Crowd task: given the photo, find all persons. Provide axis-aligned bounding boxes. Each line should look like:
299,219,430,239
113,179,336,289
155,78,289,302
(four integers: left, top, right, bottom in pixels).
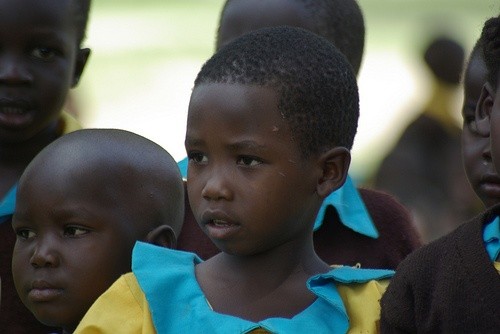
0,125,191,334
55,26,384,334
176,0,420,268
2,0,95,224
379,17,500,334
